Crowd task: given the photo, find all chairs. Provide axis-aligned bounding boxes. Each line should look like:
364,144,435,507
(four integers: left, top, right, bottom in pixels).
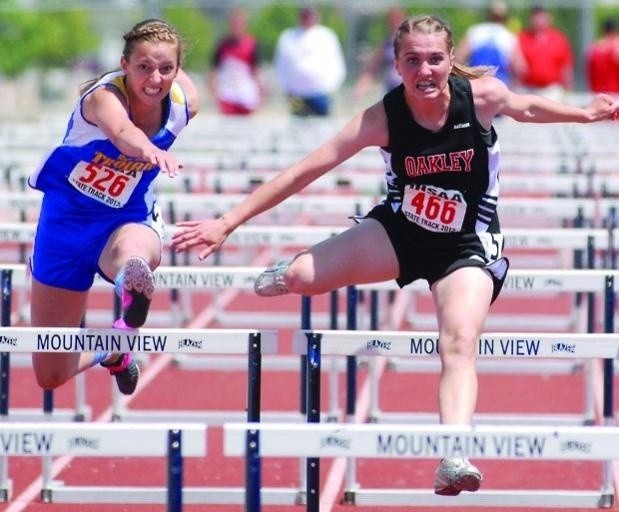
434,457,483,495
120,257,156,327
101,353,139,395
255,260,292,297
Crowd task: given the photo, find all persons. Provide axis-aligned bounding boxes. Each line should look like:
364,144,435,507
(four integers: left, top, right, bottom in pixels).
355,9,412,107
172,18,618,496
454,3,524,117
513,7,572,94
206,7,261,115
30,18,202,398
583,18,618,93
273,6,346,118
